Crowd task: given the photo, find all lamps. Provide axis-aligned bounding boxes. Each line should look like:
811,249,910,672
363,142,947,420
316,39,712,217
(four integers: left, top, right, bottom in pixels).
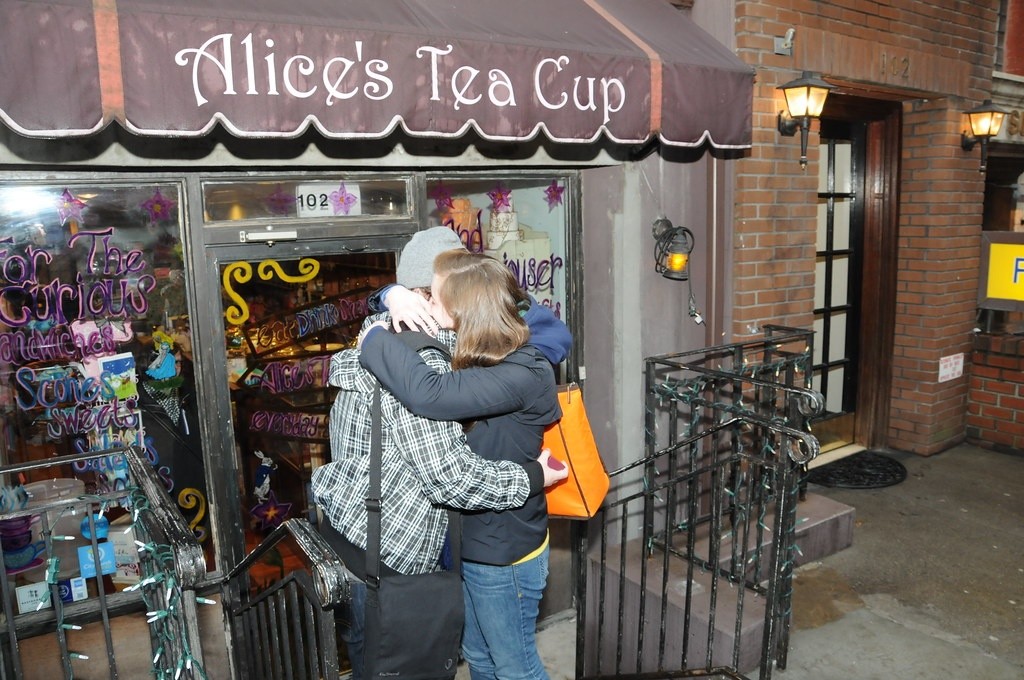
781,71,837,166
644,217,707,281
957,95,1007,152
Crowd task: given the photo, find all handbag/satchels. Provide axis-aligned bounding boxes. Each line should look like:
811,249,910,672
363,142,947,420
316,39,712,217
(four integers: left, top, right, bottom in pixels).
541,381,611,521
360,568,465,680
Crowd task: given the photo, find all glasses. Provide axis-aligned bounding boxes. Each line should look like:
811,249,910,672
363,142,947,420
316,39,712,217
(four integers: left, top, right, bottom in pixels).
175,359,183,367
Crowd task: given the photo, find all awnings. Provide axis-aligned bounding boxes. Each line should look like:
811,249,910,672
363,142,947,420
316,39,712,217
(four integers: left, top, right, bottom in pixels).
0,0,753,149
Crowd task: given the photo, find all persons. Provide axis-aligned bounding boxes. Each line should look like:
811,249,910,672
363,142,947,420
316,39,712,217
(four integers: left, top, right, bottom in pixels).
312,224,573,679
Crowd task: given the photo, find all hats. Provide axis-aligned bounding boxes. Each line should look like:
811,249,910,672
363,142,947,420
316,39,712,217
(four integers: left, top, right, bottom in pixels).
396,226,465,289
